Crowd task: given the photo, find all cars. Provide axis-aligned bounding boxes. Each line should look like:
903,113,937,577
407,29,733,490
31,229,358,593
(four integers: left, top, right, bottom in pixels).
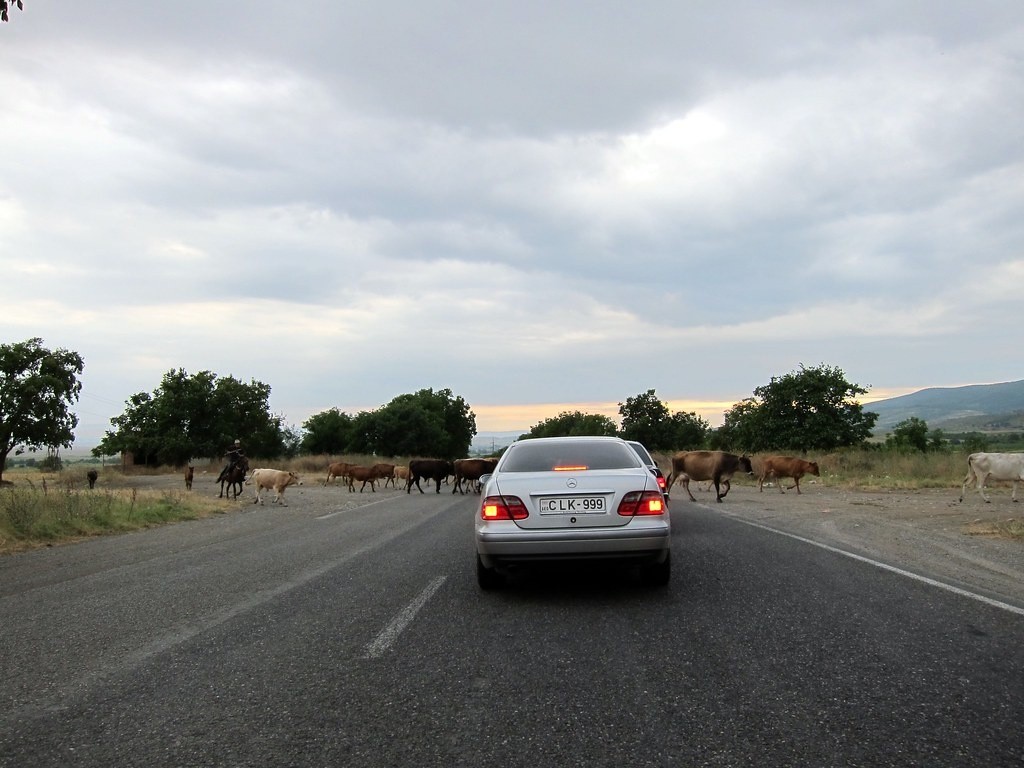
474,436,672,593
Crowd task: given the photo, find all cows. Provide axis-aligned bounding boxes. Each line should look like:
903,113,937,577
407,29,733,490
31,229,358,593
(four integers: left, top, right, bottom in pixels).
959,452,1024,503
244,468,304,507
665,451,754,503
408,459,455,494
394,466,415,490
758,456,820,494
324,463,395,493
452,457,500,496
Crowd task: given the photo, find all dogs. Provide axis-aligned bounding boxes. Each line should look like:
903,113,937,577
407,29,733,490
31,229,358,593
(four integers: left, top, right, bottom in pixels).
185,466,194,490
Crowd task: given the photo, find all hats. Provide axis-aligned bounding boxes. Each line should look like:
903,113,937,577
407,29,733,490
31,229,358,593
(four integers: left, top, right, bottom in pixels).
234,439,240,443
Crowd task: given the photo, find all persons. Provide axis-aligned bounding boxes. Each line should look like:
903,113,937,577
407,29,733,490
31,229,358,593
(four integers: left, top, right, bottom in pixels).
225,439,246,481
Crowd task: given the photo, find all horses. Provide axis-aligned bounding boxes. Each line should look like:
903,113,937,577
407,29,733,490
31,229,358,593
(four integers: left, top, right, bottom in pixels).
219,452,250,501
